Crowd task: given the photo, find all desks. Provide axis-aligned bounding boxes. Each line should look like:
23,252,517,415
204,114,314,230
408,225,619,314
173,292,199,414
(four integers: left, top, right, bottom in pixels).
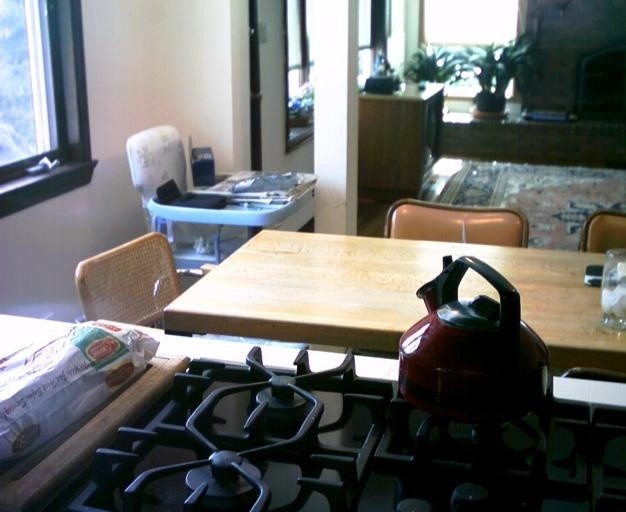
161,228,626,382
149,169,316,272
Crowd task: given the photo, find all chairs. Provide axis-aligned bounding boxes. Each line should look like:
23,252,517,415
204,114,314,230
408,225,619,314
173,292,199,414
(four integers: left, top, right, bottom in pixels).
73,231,218,338
384,198,626,251
126,125,247,273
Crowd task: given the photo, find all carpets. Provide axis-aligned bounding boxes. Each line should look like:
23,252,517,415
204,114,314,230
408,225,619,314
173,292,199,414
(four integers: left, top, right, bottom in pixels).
435,159,625,250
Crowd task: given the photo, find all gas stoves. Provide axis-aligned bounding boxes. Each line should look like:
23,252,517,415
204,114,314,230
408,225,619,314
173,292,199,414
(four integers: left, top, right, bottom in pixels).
70,342,626,512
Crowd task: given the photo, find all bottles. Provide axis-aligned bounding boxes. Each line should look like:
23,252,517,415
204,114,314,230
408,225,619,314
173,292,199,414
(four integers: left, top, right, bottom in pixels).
599,247,626,332
373,48,386,77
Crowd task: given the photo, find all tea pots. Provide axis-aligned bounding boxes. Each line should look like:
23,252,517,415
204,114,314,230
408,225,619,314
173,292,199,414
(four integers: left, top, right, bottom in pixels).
397,254,552,417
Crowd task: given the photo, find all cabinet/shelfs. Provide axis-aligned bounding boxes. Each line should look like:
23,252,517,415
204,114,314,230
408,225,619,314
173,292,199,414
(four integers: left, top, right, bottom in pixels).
356,81,445,203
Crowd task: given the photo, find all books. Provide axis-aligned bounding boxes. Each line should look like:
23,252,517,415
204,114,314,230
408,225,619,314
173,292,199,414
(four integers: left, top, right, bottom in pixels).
205,169,317,209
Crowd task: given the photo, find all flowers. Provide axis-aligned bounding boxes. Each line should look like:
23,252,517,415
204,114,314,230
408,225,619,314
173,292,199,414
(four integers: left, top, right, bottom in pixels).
287,81,314,120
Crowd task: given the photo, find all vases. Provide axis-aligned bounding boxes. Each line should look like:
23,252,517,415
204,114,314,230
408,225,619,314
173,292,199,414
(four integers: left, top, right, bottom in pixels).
289,117,310,127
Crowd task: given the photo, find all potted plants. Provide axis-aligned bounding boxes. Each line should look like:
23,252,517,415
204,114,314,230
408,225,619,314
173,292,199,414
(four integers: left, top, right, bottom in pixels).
468,31,540,121
